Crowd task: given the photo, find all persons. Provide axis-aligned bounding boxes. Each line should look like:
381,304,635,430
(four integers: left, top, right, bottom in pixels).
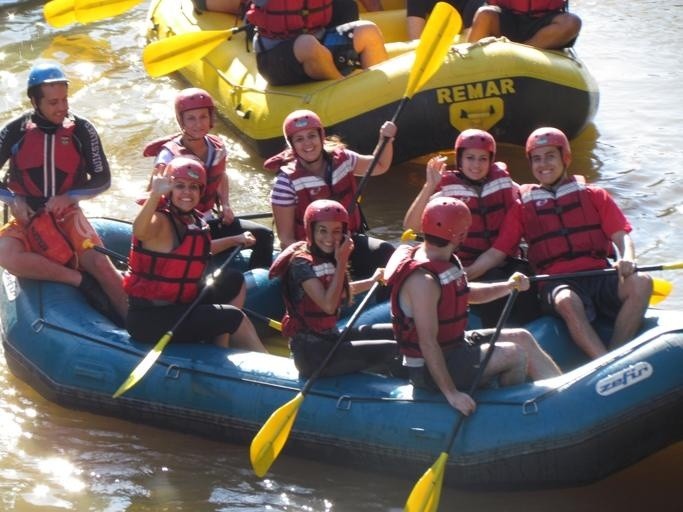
268,105,396,280
146,85,277,274
277,197,402,378
387,193,562,417
0,64,129,324
459,125,655,360
196,0,388,86
402,128,538,330
126,155,271,357
406,0,582,49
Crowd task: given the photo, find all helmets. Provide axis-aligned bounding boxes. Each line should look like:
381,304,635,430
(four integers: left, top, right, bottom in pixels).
454,129,496,166
304,199,350,247
420,196,471,246
525,126,572,166
28,63,70,87
162,156,206,197
175,87,214,128
283,110,326,145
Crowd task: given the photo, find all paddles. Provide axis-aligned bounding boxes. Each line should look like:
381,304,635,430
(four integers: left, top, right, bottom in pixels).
349,2,463,212
113,234,249,399
402,282,520,512
401,229,671,305
141,23,254,80
42,1,77,27
73,1,145,24
249,230,417,478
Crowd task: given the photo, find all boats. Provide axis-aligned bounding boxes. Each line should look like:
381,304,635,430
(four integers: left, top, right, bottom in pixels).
0,214,682,493
150,2,598,158
